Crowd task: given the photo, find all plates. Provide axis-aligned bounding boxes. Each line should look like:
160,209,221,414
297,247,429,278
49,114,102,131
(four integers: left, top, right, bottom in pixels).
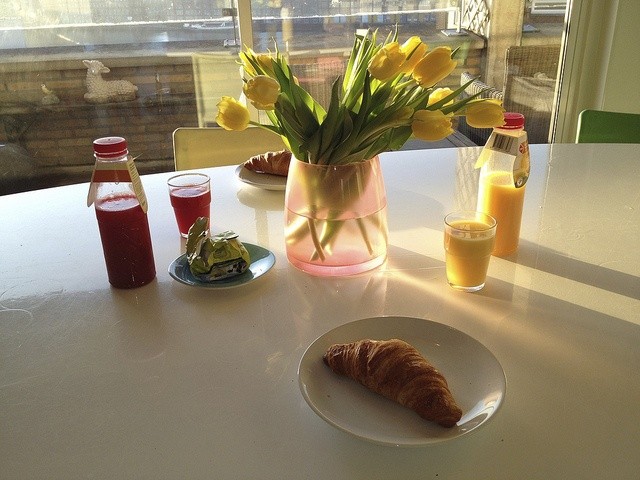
297,315,507,449
168,242,276,290
233,164,286,191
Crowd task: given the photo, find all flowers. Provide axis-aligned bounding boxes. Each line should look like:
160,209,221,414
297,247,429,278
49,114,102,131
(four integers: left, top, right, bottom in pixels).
216,23,506,262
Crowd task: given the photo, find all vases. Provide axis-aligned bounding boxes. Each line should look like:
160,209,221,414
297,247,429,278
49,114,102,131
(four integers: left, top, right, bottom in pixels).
283,153,389,278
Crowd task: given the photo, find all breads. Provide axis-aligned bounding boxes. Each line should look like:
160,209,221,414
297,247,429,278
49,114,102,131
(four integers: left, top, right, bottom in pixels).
239,148,292,177
323,339,462,428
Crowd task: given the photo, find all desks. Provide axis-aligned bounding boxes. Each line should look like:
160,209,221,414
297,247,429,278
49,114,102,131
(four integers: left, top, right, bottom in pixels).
399,130,479,148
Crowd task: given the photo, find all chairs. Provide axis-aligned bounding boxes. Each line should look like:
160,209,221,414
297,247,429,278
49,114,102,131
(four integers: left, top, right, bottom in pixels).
576,109,640,144
173,126,289,171
457,73,502,145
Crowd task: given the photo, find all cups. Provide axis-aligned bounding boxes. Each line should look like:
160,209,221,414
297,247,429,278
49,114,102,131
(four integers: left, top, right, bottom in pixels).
444,210,497,292
167,173,211,238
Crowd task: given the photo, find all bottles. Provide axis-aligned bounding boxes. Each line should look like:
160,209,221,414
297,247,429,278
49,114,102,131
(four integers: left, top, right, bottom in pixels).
93,137,156,289
475,112,531,258
